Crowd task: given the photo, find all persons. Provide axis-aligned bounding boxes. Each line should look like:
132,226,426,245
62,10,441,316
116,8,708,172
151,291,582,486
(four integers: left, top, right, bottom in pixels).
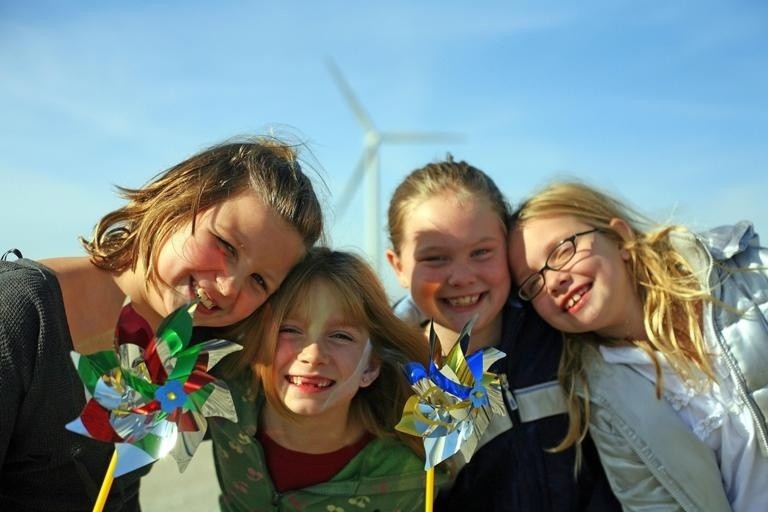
0,133,323,512
179,241,450,512
382,156,623,512
503,178,768,511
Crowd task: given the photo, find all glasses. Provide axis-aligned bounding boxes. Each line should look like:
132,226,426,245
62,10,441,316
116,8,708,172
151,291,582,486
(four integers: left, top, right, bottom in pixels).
514,226,602,302
